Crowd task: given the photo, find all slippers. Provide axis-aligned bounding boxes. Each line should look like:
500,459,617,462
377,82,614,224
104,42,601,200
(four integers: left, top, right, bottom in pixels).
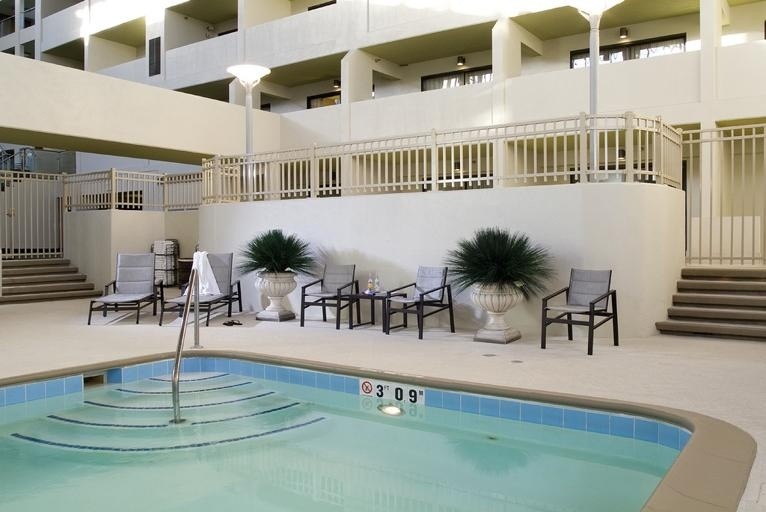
223,320,242,326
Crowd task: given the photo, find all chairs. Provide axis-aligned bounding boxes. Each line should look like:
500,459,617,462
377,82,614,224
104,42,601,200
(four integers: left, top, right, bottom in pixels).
296,260,458,342
540,266,620,357
87,250,243,327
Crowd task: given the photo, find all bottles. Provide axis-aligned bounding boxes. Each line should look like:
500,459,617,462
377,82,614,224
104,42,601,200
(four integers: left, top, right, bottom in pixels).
374,272,380,291
368,273,373,291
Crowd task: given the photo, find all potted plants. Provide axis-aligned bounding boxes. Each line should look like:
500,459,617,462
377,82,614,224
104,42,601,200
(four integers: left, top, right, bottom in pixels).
232,228,324,324
438,226,559,345
